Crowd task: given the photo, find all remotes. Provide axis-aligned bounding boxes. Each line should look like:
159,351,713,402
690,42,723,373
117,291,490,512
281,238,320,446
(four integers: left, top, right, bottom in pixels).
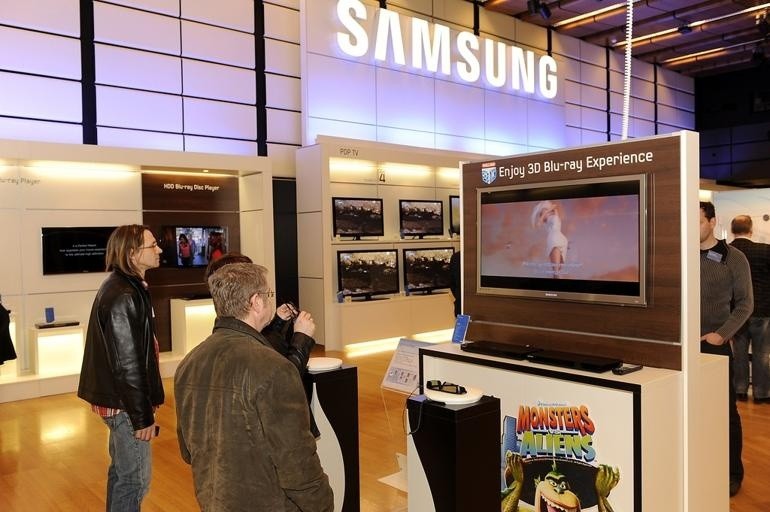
612,364,645,375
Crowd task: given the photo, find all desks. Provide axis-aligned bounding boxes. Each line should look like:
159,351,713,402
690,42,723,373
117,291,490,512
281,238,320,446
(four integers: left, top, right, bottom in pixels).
170,297,220,356
29,326,86,373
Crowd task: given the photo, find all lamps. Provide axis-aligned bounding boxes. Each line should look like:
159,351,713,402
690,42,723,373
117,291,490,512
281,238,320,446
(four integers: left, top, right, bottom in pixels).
756,11,770,40
751,42,764,60
527,0,551,20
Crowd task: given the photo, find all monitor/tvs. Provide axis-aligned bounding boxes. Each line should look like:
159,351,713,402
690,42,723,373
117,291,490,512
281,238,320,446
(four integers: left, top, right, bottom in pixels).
404,247,455,294
449,194,460,234
475,171,650,308
40,225,116,275
332,196,384,241
159,223,228,269
399,198,444,238
337,248,399,301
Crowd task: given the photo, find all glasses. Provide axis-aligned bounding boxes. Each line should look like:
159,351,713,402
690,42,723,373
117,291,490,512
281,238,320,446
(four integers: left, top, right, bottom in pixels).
259,289,274,298
285,300,300,317
137,240,158,250
426,379,467,395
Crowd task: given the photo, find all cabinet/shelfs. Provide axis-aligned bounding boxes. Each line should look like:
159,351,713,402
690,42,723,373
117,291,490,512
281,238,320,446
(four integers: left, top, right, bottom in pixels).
296,135,498,352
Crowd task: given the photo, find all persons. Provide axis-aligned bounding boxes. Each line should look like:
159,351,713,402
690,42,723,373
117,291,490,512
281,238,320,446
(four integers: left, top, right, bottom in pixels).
0,296,17,370
177,231,224,266
529,199,569,280
200,250,324,440
75,220,167,512
727,210,770,404
697,198,756,497
171,261,336,512
447,248,464,317
